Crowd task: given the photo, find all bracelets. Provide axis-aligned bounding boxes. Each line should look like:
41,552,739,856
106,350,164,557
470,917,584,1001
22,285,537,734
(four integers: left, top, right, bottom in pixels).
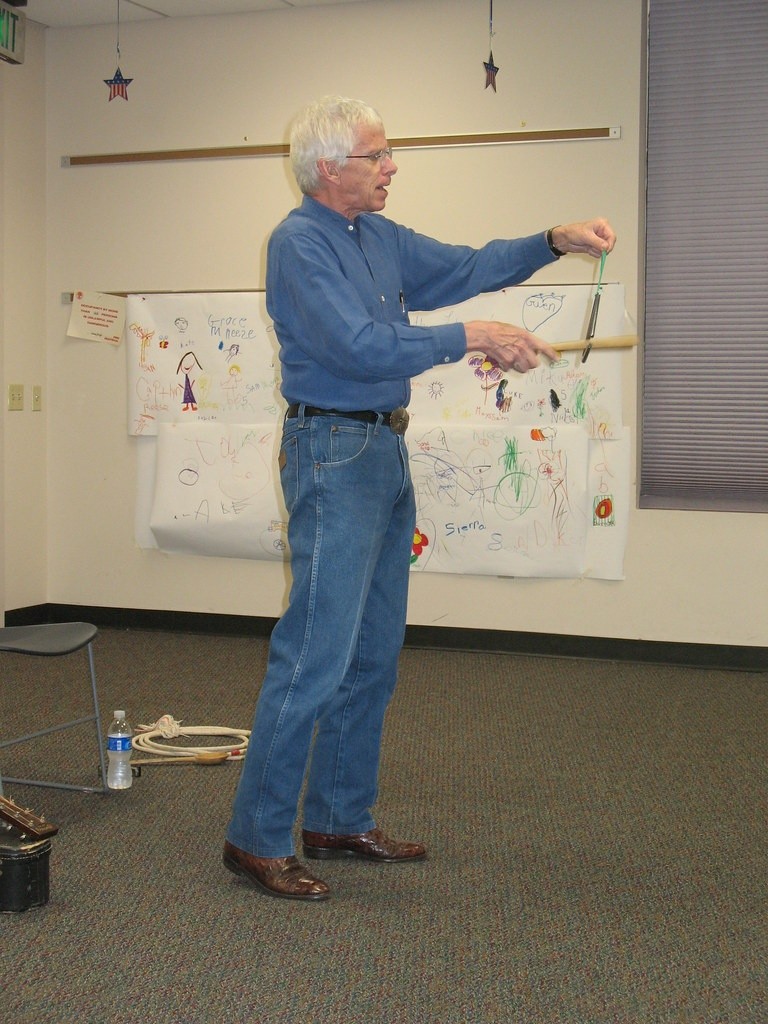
547,226,565,255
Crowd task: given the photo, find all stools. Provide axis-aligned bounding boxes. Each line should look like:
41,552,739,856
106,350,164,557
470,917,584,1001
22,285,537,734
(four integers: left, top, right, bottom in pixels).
0,622,107,793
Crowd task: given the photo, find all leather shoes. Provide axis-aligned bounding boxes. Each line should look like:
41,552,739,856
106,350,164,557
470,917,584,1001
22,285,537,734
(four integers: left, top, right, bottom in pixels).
301,826,427,863
221,836,331,901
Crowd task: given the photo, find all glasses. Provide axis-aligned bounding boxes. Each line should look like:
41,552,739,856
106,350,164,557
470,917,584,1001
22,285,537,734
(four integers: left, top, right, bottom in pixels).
346,146,393,161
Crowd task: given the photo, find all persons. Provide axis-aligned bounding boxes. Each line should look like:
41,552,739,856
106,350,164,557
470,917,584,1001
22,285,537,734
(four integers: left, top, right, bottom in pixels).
221,98,616,902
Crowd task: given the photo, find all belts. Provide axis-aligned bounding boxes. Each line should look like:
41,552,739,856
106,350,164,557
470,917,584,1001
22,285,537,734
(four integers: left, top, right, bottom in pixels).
287,402,410,434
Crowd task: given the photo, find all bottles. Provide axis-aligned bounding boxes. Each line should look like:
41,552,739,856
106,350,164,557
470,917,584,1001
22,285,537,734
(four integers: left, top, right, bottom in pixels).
107,710,132,790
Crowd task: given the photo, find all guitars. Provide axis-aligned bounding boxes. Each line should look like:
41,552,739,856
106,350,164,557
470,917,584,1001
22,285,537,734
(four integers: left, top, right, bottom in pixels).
1,792,60,840
